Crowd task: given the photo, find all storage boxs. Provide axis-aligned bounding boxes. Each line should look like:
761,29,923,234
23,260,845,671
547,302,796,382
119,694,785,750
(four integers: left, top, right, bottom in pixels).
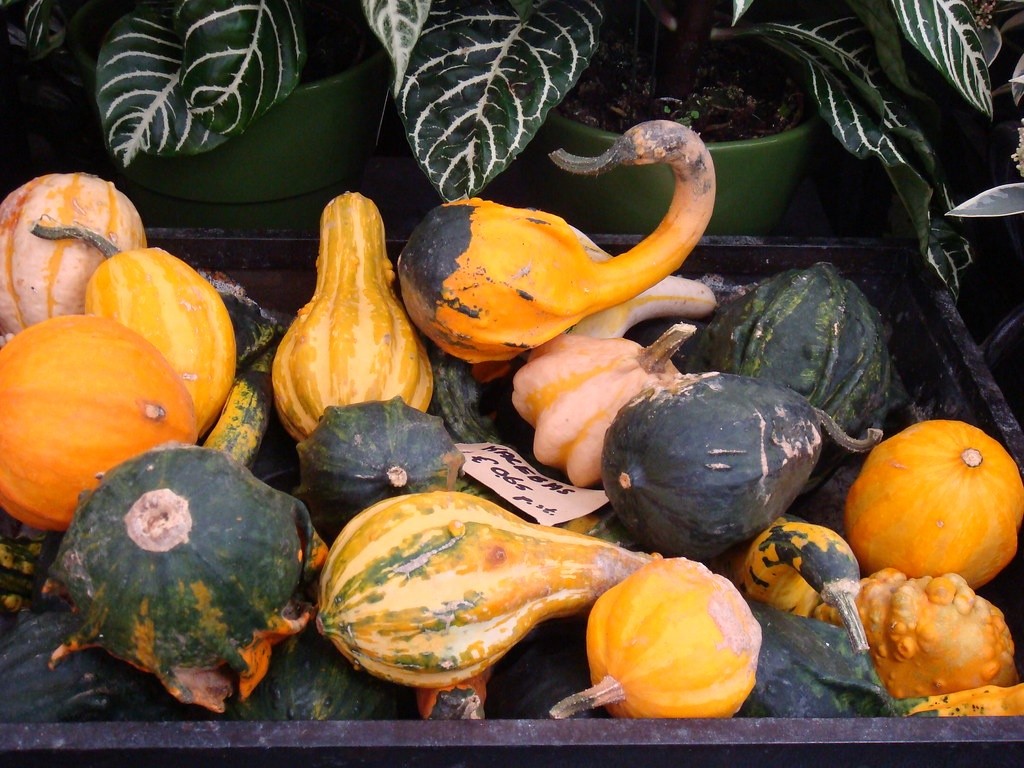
0,225,1024,768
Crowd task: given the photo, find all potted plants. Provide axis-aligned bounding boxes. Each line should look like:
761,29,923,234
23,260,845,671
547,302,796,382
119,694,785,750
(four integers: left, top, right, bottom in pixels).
0,0,1024,312
